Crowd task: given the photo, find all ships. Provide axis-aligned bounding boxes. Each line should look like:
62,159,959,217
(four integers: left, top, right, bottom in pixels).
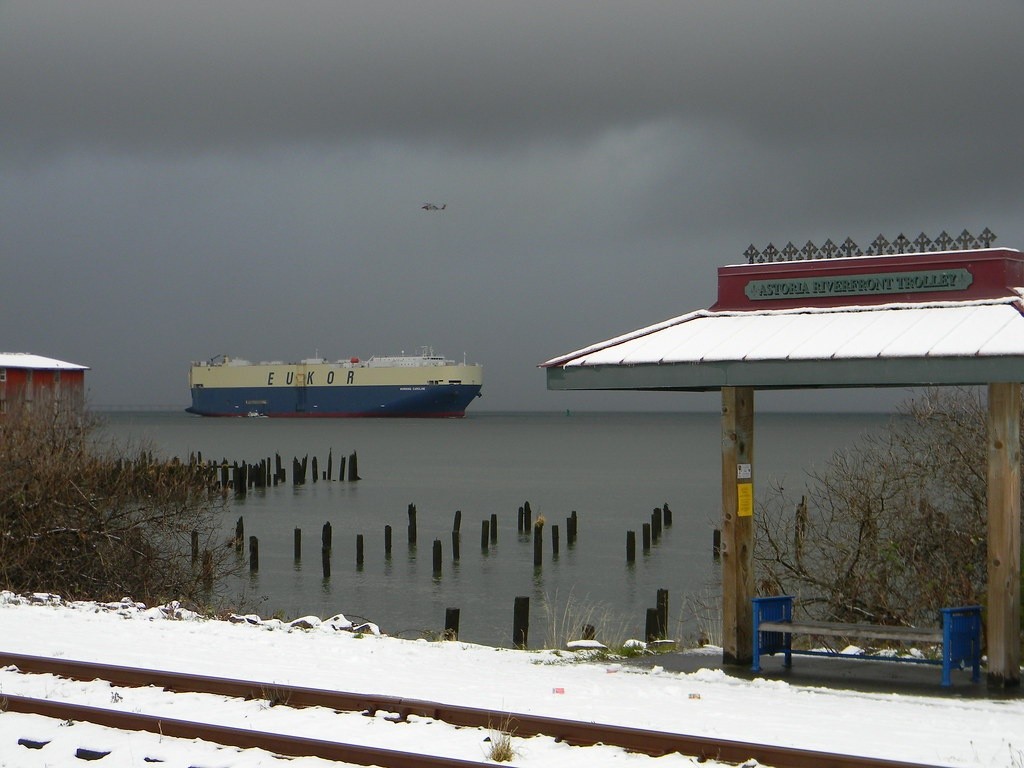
184,345,487,419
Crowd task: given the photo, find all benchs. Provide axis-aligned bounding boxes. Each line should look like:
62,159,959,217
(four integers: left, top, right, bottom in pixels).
749,594,986,687
